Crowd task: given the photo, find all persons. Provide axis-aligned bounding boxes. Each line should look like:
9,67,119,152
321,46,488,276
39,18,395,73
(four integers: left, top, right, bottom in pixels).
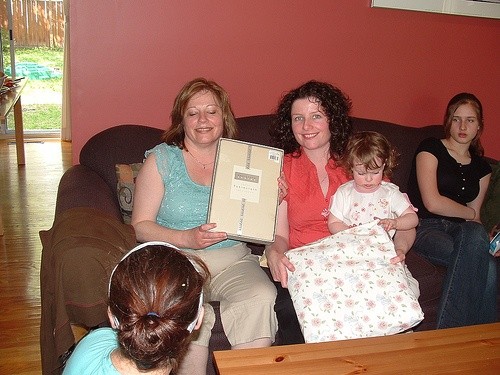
61,240,210,375
131,78,279,375
409,92,500,330
327,131,420,240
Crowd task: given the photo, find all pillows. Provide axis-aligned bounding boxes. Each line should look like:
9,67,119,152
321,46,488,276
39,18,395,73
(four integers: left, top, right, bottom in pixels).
115,163,143,224
284,222,424,344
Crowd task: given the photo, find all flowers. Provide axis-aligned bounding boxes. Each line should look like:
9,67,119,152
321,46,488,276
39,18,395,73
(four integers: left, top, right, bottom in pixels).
0,72,25,105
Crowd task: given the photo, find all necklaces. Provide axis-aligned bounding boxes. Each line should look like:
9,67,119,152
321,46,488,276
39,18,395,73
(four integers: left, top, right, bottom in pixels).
185,148,214,171
319,174,327,185
265,80,416,344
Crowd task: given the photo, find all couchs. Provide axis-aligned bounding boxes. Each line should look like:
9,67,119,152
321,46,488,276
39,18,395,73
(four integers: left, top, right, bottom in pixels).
39,114,500,375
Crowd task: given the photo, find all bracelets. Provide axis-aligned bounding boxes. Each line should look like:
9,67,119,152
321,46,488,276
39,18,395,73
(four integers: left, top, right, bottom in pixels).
465,207,477,222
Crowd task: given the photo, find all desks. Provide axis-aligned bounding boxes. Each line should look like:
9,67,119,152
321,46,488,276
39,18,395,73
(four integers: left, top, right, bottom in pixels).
0,77,28,165
212,322,500,375
